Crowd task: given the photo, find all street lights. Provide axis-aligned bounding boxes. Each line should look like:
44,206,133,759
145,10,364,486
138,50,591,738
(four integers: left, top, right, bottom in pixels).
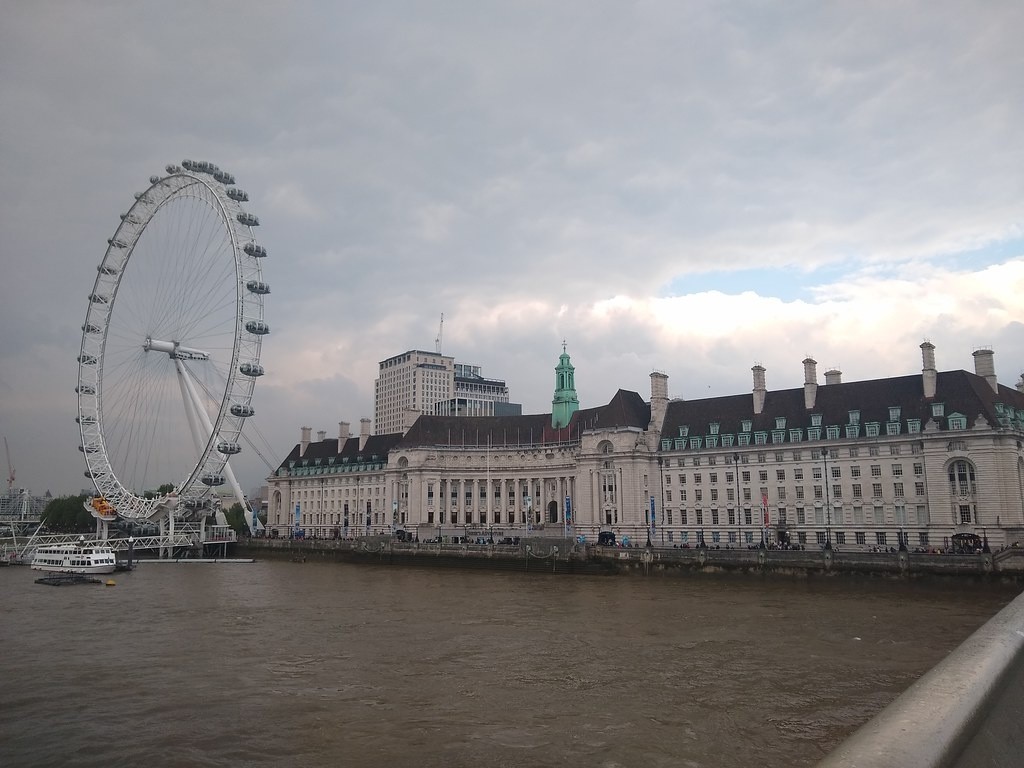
488,524,494,543
463,525,469,543
437,524,442,543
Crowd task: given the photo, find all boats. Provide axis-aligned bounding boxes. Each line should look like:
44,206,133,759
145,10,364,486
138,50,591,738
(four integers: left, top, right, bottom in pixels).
30,544,117,574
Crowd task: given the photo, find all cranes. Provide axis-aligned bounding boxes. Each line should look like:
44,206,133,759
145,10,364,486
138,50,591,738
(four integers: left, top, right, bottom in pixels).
3,435,16,492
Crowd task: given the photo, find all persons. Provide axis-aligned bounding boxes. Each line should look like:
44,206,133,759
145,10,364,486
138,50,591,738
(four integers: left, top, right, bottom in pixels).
696,544,699,548
1001,540,1019,551
423,537,438,543
868,545,896,552
932,548,941,552
680,543,689,548
469,539,488,545
755,540,805,550
